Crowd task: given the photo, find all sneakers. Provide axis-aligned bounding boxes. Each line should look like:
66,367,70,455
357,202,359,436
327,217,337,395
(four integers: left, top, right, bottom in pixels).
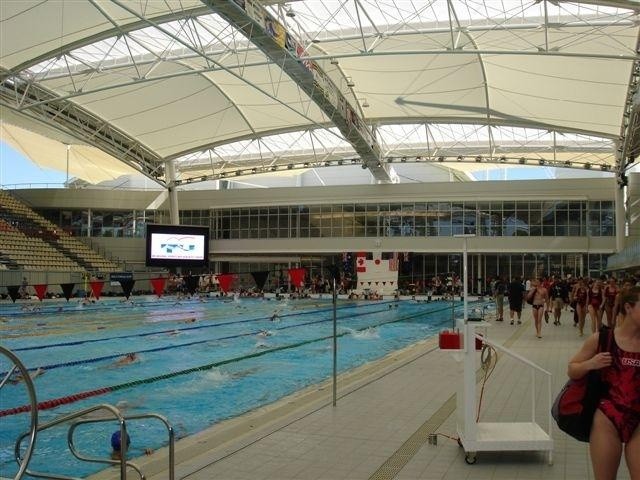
496,318,521,325
554,321,560,326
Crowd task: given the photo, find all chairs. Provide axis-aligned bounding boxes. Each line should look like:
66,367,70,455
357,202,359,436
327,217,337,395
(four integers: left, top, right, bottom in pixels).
0,186,132,271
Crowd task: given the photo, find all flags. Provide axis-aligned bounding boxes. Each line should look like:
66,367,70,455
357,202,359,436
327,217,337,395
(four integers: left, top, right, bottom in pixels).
356,251,367,272
341,251,353,272
388,252,400,271
372,251,383,270
402,251,413,272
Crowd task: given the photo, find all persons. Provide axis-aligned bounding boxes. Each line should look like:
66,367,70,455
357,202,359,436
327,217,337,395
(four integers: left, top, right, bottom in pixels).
3,367,47,386
170,273,462,310
21,277,139,305
486,274,637,338
99,350,139,370
108,428,154,469
565,285,640,480
37,392,145,430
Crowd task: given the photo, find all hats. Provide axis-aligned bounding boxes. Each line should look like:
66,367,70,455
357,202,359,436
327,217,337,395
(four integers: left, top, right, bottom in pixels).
111,431,130,446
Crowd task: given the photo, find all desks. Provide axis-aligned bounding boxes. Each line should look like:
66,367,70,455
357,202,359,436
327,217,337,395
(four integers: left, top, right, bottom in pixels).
456,318,492,350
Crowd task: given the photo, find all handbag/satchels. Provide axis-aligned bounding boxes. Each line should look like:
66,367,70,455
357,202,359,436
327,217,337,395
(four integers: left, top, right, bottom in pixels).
527,288,537,305
552,369,597,443
